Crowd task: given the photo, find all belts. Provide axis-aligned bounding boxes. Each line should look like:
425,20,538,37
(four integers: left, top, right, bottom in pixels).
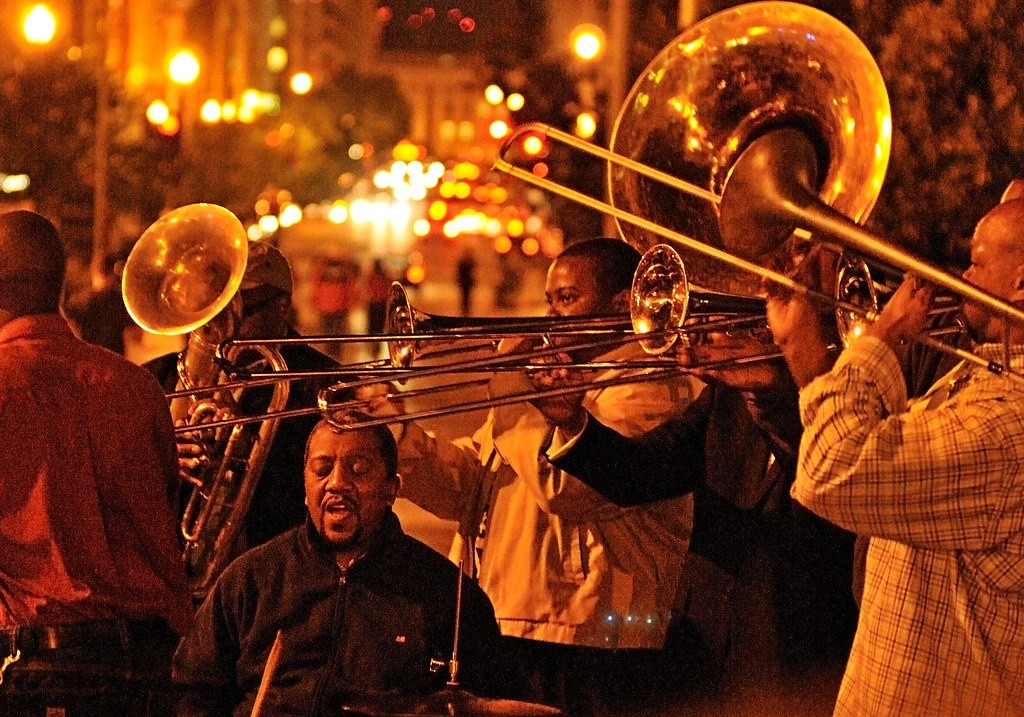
1,621,171,653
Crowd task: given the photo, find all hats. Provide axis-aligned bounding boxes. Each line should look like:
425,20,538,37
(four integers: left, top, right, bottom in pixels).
238,239,293,296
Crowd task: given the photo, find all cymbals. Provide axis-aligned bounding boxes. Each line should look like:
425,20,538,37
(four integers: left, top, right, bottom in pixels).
341,671,561,715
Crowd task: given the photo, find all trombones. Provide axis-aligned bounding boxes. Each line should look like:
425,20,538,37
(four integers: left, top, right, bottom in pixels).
492,118,1024,385
168,241,961,438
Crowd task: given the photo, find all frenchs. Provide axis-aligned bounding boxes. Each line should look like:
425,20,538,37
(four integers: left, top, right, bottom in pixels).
609,4,896,297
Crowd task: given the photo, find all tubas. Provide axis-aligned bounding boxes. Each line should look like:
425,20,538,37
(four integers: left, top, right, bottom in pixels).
120,193,288,596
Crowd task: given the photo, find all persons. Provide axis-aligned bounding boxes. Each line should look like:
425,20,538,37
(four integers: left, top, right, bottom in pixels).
140,242,361,588
363,260,390,354
1,211,197,717
78,254,132,356
457,251,476,317
315,253,360,361
677,161,1024,468
523,284,859,716
354,238,695,650
148,410,506,716
765,198,1024,716
